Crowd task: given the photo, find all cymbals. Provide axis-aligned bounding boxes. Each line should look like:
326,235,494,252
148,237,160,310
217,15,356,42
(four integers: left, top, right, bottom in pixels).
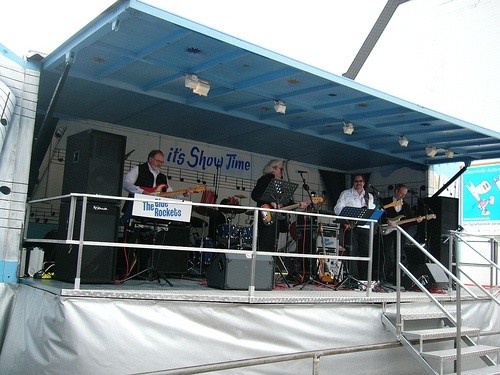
244,211,255,215
187,217,207,229
194,207,220,218
218,203,246,214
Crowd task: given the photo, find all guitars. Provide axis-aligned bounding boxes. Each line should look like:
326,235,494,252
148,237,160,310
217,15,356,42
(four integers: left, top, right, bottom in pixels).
261,194,324,225
378,214,436,235
317,221,335,283
138,183,210,199
343,200,403,231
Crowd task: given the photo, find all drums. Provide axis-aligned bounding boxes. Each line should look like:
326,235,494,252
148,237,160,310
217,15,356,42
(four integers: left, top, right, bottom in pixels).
216,223,237,246
194,237,217,264
236,225,254,240
231,243,252,250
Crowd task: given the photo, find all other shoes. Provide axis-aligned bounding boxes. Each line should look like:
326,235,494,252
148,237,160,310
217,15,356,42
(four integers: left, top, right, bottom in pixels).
158,272,169,278
140,271,150,279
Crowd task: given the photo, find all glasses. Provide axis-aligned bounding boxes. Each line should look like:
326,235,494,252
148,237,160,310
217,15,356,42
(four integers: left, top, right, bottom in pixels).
276,167,284,172
354,180,364,183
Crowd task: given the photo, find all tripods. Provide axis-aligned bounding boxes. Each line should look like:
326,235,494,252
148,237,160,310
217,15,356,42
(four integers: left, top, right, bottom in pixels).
292,173,375,291
181,211,210,278
120,219,174,287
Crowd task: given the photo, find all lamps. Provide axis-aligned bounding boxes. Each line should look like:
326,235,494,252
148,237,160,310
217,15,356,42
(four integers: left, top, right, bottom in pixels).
184,73,210,96
398,136,409,147
342,122,354,135
273,100,287,113
426,146,438,157
446,151,454,158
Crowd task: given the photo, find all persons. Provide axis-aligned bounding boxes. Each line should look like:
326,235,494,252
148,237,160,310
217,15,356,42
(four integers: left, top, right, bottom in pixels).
334,174,376,290
116,149,194,282
380,183,423,286
251,159,306,259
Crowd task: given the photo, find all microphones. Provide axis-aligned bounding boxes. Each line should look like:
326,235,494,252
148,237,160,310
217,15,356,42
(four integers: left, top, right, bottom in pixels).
295,170,308,173
369,184,378,191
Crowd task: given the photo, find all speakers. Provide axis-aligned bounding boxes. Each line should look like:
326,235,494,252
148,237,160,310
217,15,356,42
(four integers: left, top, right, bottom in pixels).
404,196,459,292
129,223,189,275
206,253,275,291
55,129,127,284
